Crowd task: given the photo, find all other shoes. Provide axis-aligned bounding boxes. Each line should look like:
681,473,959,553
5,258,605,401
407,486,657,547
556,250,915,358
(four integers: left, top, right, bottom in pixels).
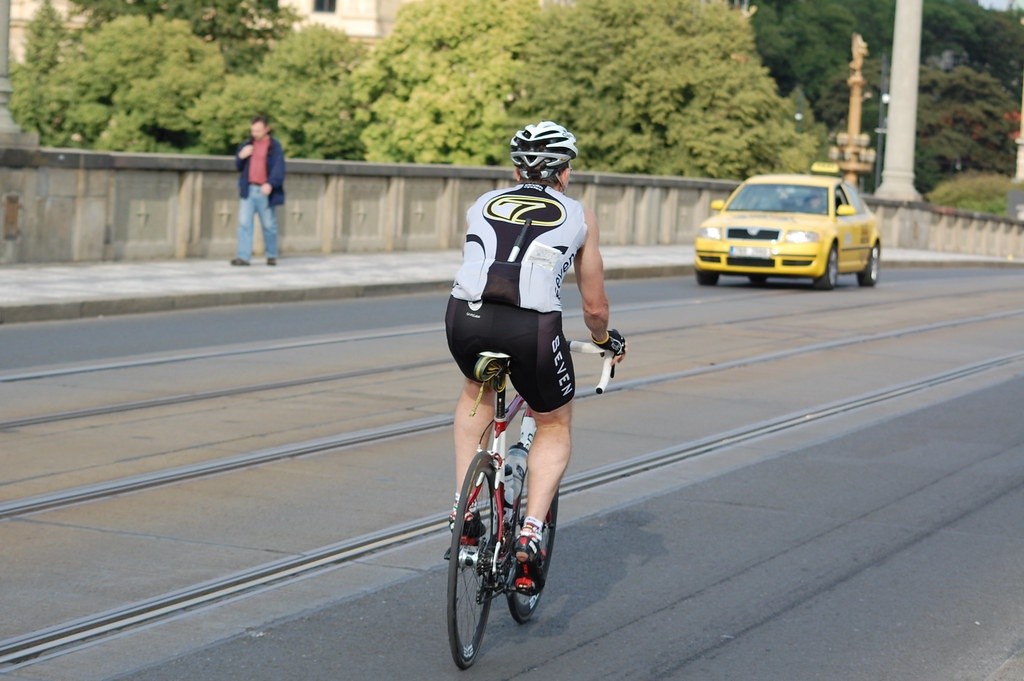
232,258,249,266
267,256,277,265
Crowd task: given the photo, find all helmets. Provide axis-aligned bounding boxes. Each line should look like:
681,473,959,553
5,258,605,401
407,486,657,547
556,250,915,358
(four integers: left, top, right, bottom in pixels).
509,121,579,181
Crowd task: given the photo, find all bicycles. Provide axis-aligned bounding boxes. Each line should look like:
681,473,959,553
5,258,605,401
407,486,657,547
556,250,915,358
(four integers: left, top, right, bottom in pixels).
443,341,615,670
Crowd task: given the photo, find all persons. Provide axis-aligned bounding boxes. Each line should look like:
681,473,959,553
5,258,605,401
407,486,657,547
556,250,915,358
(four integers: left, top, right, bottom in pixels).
799,189,827,215
445,120,626,595
231,117,285,267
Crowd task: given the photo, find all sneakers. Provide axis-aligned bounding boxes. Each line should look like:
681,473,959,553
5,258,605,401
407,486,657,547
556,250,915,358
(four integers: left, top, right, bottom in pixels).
512,531,546,597
447,512,486,563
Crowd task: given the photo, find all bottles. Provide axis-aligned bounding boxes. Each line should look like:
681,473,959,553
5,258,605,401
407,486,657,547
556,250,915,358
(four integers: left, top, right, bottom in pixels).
505,441,528,500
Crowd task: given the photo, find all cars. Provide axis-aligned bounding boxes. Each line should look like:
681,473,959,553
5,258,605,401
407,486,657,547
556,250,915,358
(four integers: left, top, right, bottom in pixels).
696,161,880,290
